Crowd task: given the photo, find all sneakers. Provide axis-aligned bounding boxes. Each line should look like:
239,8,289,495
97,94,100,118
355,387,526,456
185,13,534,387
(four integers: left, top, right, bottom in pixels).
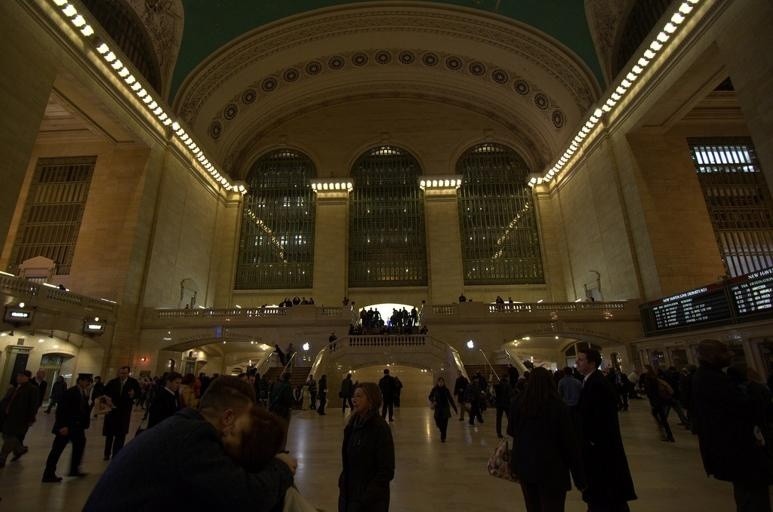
317,409,326,416
389,419,394,422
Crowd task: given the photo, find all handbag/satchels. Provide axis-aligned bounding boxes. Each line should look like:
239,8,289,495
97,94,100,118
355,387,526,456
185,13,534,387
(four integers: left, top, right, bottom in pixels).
134,426,146,438
484,437,524,486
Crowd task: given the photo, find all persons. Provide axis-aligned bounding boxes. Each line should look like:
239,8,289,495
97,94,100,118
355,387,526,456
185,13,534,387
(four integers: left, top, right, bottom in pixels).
329,333,337,352
57,284,64,289
429,339,771,512
277,295,430,339
261,304,267,308
337,381,395,512
80,374,318,512
494,296,504,312
458,293,466,303
508,296,513,312
273,343,296,368
1,367,403,483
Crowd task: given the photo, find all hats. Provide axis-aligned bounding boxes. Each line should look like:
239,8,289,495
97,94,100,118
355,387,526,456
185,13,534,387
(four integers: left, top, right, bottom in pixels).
16,369,32,377
79,372,94,381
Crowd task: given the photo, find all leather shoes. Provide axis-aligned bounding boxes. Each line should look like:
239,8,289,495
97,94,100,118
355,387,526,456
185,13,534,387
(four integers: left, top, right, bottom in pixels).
68,469,88,477
10,445,28,463
40,472,63,483
